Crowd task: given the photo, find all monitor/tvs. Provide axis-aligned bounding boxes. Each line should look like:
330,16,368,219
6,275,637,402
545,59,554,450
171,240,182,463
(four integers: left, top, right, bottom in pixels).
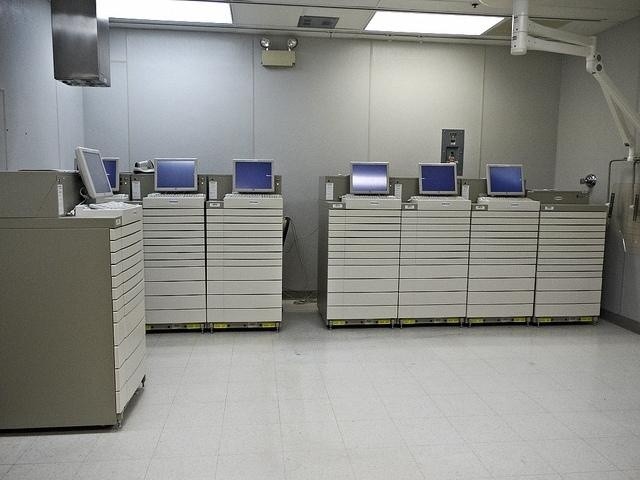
350,161,389,194
75,147,274,204
419,163,457,195
486,163,525,196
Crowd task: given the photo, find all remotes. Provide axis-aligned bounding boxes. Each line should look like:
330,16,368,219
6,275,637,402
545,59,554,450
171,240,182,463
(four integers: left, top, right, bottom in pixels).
326,183,333,200
462,185,470,200
274,175,282,194
395,184,402,199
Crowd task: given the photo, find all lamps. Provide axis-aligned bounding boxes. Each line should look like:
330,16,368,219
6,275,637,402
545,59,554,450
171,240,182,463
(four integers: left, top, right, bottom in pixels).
258,36,300,69
